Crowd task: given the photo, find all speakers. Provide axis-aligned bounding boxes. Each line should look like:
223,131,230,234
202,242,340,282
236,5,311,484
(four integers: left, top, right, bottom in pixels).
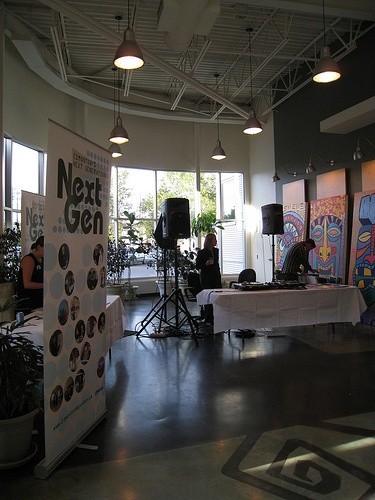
261,204,284,235
153,198,190,250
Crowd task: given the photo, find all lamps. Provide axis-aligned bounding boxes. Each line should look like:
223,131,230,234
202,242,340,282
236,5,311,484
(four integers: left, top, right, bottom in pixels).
273,166,297,182
108,68,130,145
306,152,334,174
108,68,123,158
352,136,375,161
312,0,342,83
114,0,144,69
242,27,264,135
210,74,227,161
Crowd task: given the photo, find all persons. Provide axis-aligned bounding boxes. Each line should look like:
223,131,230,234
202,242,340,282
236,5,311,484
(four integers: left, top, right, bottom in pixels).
196,234,223,288
17,235,44,310
281,239,319,281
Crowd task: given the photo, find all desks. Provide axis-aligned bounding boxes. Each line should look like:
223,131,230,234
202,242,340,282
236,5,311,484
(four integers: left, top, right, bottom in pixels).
0,295,129,362
196,283,368,345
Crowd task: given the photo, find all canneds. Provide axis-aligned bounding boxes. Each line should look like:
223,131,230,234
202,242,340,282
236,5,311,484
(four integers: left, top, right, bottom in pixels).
16,312,24,326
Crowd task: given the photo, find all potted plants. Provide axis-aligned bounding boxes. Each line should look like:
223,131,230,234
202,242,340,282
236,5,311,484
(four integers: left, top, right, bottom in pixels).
107,212,225,302
0,222,44,469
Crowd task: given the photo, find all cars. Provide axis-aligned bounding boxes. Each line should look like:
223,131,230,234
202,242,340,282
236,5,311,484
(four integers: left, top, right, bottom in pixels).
124,247,162,267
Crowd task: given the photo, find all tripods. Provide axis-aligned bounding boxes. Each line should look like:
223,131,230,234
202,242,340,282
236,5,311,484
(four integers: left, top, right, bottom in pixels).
135,240,198,347
